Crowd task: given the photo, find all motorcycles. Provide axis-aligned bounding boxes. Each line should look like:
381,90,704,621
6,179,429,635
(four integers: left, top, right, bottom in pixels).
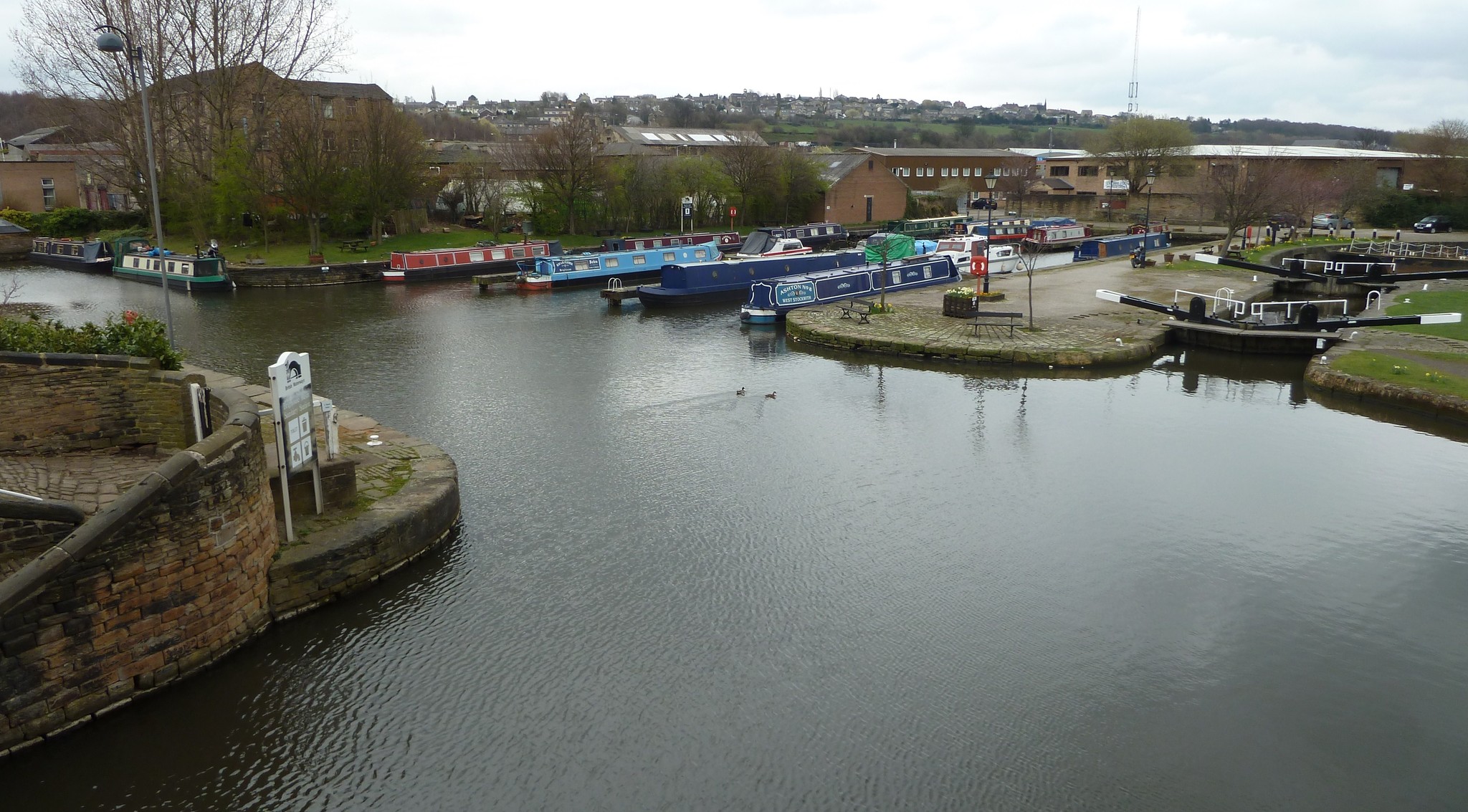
1129,246,1142,268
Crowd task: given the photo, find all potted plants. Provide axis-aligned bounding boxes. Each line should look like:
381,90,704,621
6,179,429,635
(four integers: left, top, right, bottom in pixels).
1164,251,1175,264
246,253,252,262
1301,231,1310,238
1230,244,1240,250
1261,236,1271,245
1246,242,1255,248
251,254,265,264
1178,253,1191,261
1145,258,1157,267
308,249,324,264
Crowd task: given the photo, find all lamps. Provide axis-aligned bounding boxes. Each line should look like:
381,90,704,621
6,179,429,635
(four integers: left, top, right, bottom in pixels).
1001,162,1005,165
900,167,903,170
924,162,928,166
851,204,855,208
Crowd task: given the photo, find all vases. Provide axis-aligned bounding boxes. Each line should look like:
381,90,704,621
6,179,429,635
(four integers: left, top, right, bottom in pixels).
942,294,979,320
1378,236,1394,241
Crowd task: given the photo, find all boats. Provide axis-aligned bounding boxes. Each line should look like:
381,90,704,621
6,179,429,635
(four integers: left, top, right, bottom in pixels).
636,216,1093,324
29,235,116,275
514,240,725,290
736,232,813,258
378,240,566,283
1072,220,1172,262
740,222,850,247
598,230,744,255
112,235,227,291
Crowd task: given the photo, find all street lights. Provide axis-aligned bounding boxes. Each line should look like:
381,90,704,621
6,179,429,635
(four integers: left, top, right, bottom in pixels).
93,25,176,359
1139,168,1157,268
1108,168,1116,222
676,145,688,156
983,168,999,293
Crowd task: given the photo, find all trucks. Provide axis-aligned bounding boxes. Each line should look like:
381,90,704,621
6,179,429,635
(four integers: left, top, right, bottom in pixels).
434,179,544,221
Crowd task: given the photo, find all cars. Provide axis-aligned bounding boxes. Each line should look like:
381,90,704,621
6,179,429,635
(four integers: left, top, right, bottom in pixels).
1313,213,1353,230
1413,215,1455,234
972,197,997,210
1269,214,1306,228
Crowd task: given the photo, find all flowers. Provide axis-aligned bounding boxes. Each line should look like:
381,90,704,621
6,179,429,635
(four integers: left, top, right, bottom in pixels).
1380,234,1392,236
946,287,974,298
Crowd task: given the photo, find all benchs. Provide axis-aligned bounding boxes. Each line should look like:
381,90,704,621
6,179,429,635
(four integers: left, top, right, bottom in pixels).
1221,255,1246,260
1195,246,1214,255
836,296,875,325
966,311,1024,338
595,228,615,237
1277,232,1300,244
350,245,370,249
336,245,356,247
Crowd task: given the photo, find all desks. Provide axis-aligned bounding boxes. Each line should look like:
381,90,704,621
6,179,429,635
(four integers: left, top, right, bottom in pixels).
340,239,367,253
1224,250,1244,261
